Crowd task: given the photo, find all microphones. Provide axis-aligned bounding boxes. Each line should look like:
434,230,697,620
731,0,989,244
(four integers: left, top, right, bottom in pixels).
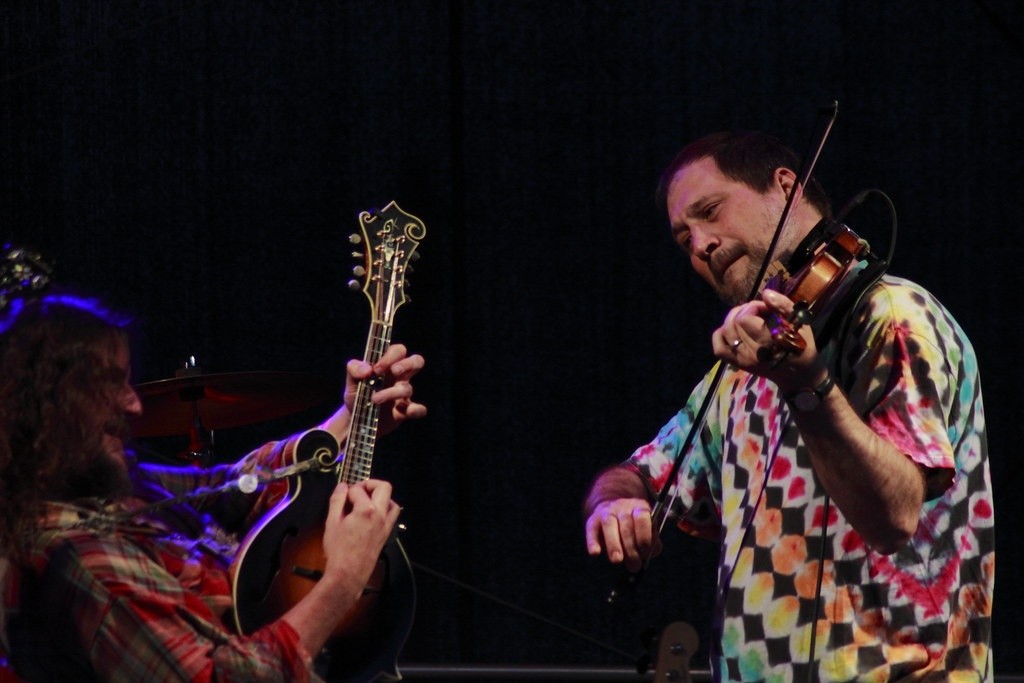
786,187,871,276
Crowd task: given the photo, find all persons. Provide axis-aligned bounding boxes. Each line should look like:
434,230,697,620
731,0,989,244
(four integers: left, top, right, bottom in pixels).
0,295,427,683
582,131,996,683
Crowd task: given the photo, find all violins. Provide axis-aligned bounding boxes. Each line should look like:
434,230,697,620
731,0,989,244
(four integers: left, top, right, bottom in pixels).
756,226,869,364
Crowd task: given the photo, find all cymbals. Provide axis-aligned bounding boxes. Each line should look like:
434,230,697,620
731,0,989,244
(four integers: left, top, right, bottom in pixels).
120,362,343,438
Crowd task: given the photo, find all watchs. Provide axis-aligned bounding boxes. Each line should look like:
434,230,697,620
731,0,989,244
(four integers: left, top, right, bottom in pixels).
785,374,834,412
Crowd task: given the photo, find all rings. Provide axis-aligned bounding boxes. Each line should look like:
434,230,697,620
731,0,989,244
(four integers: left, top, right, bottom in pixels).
731,339,743,352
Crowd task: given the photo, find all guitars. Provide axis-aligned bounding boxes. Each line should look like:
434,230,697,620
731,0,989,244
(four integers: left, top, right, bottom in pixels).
228,200,428,683
